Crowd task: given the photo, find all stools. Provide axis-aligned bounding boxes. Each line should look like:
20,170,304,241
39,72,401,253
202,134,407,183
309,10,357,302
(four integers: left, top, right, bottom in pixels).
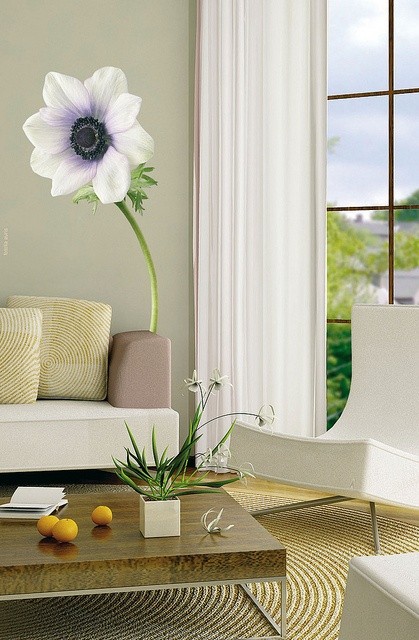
339,555,419,638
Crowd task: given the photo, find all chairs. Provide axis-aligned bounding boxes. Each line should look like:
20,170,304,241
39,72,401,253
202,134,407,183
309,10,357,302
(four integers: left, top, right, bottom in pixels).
229,304,419,554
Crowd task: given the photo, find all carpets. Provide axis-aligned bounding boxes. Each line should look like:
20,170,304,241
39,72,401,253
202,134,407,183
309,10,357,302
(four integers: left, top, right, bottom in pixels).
0,482,419,640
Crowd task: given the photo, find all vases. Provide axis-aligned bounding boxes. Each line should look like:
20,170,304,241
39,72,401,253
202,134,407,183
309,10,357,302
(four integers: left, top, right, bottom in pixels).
138,493,180,539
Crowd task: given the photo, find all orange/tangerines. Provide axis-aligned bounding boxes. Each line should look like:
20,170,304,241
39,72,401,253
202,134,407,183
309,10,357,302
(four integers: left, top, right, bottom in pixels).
92,506,112,524
37,515,58,537
54,519,78,542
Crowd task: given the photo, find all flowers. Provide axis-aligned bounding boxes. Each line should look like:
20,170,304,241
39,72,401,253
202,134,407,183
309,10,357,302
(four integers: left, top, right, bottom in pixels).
198,506,234,540
20,64,173,333
110,364,279,495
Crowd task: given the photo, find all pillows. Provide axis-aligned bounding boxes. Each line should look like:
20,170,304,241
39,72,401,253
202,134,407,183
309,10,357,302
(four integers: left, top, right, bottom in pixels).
1,308,43,404
9,296,112,401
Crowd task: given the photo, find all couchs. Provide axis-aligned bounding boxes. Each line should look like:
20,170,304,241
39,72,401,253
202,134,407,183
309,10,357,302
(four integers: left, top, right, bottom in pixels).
1,330,181,470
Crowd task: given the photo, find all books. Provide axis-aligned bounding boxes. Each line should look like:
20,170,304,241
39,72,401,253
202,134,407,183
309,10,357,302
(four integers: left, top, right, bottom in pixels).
0,487,69,523
